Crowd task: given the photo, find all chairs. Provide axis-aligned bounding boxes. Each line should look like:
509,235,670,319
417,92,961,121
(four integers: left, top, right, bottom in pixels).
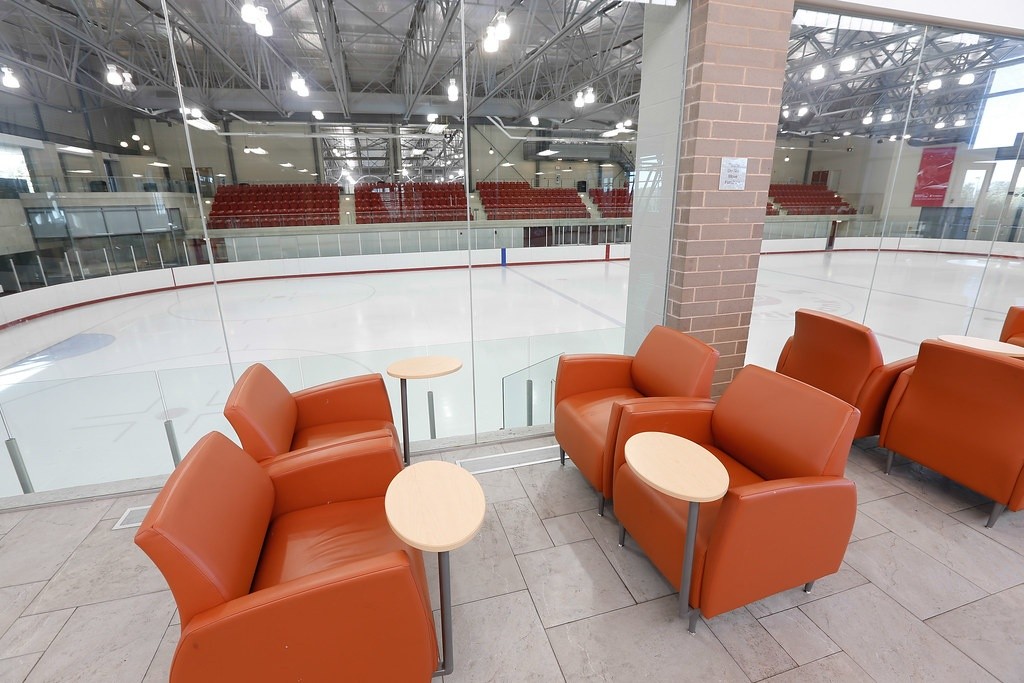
766,184,856,216
206,180,633,230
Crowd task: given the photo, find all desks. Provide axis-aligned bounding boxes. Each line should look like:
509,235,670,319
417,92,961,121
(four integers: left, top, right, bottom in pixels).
937,334,1024,358
386,354,462,464
385,458,487,676
624,432,730,622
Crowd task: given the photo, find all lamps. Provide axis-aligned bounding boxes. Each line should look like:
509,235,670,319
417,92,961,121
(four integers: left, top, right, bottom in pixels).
448,38,458,103
0,63,20,89
106,59,137,92
119,132,151,151
574,82,595,109
483,6,510,53
489,120,494,155
243,121,251,154
290,42,309,98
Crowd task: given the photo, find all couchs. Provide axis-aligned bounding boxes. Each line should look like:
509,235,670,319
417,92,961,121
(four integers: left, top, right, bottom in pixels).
999,306,1024,348
776,307,916,437
554,322,719,518
224,363,405,464
611,365,860,636
134,432,439,683
878,339,1024,530
